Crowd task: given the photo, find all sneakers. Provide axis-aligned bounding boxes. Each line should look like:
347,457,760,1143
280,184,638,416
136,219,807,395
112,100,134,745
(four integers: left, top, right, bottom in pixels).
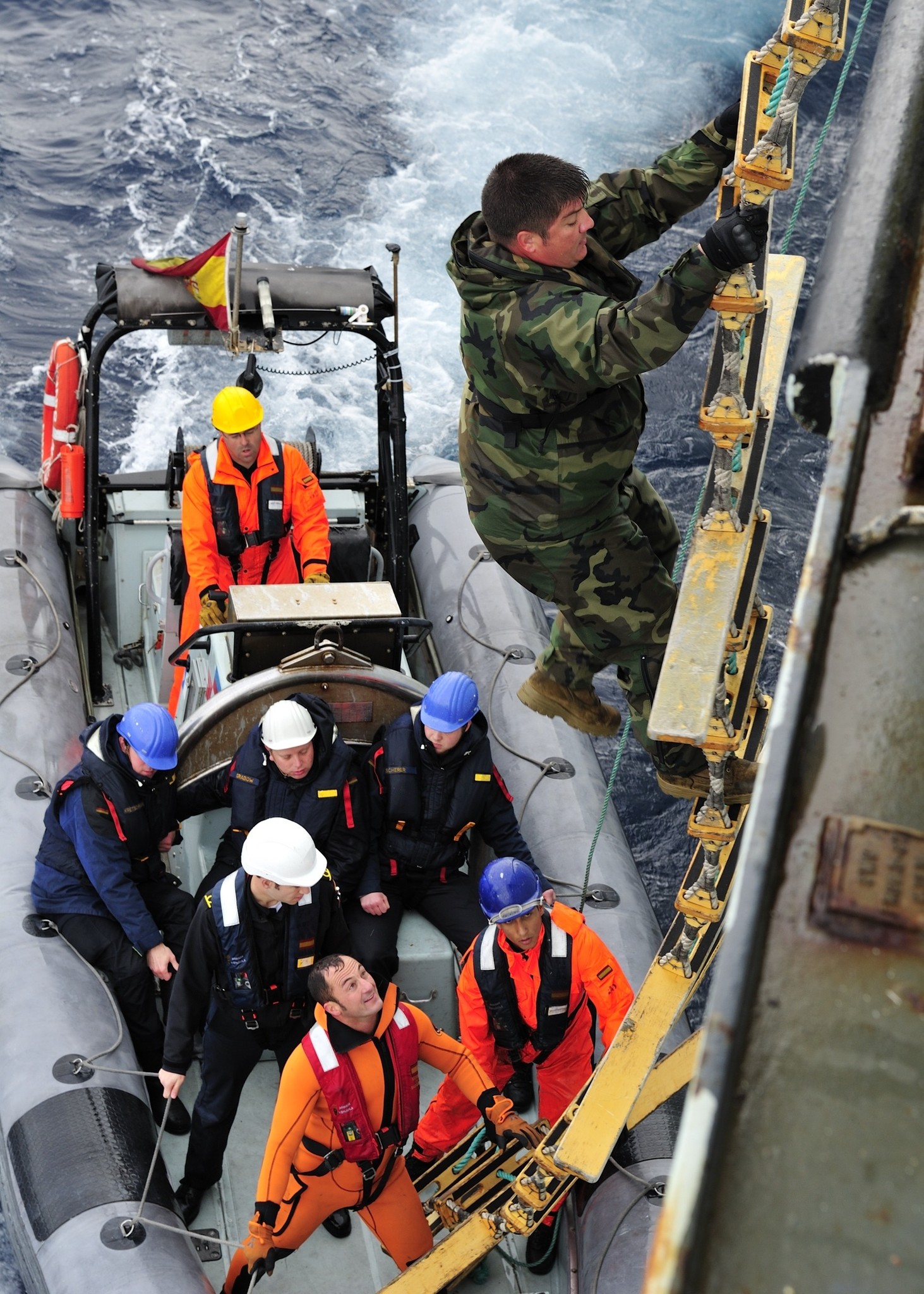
656,752,760,804
515,669,621,736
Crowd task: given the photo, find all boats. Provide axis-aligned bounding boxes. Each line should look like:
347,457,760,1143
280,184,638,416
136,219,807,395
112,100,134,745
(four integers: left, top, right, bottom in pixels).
1,213,703,1294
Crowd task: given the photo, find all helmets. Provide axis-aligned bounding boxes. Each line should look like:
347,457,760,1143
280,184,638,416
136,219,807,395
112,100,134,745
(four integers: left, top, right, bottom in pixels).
477,856,543,924
420,671,480,733
258,700,317,750
116,701,179,771
241,817,328,887
211,386,264,434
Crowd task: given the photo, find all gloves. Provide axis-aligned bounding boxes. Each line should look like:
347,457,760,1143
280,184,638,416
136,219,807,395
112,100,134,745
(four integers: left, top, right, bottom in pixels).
303,572,331,584
240,1200,280,1276
485,1093,543,1156
699,201,769,272
198,589,229,628
714,98,740,138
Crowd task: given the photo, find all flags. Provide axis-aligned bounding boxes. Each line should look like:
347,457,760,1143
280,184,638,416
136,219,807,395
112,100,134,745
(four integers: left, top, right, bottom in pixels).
131,231,234,333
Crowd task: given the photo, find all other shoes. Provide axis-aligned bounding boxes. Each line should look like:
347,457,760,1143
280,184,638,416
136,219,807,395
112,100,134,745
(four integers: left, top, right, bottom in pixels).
500,1062,536,1115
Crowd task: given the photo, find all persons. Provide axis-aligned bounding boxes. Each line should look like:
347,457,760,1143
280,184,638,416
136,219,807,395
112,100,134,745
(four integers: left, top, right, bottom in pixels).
219,954,544,1294
31,673,557,1229
403,856,636,1276
167,385,331,720
446,101,768,805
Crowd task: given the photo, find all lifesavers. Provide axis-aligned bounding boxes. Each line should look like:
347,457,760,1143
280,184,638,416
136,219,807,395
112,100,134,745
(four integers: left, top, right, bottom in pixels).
40,337,82,488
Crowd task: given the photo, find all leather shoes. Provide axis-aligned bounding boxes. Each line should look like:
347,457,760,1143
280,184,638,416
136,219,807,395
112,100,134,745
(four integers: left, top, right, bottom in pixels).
174,1183,210,1227
322,1208,352,1238
525,1230,559,1275
148,1081,192,1135
